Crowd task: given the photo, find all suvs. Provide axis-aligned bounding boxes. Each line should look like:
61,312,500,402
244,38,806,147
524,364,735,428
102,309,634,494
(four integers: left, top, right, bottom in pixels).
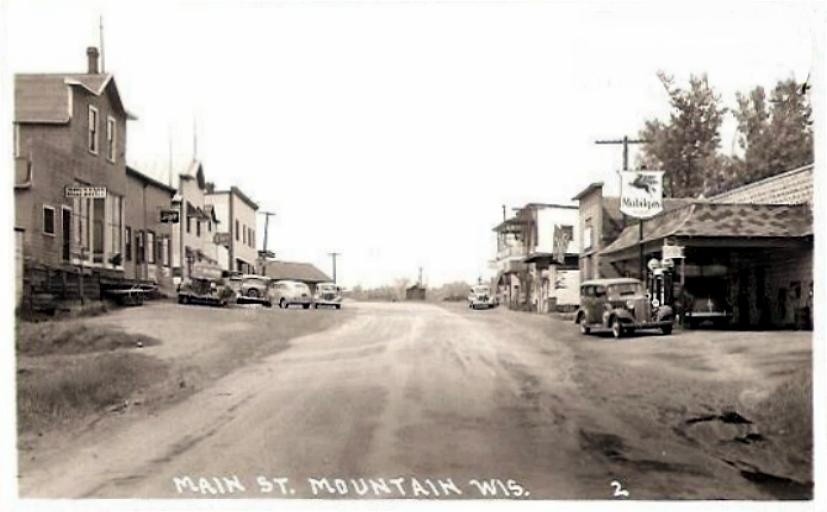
673,275,732,331
571,274,673,339
266,276,315,312
173,258,235,308
465,282,500,310
233,270,273,310
311,279,345,312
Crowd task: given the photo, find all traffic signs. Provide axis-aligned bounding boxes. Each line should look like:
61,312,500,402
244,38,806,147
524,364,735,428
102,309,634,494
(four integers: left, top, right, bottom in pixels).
61,184,108,200
